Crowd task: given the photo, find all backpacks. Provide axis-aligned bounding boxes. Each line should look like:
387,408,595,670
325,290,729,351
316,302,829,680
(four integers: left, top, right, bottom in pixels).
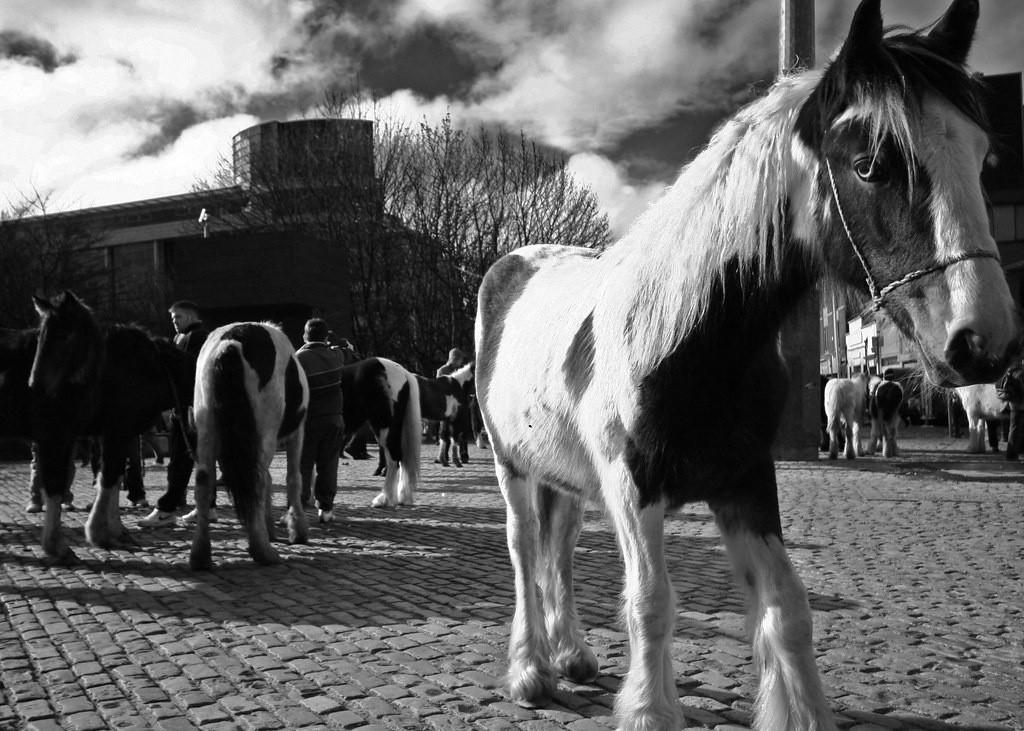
995,367,1020,400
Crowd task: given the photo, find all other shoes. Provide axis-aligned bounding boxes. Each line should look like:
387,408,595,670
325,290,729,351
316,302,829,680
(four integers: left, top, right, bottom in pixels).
318,508,335,523
461,453,469,463
61,502,74,510
992,447,998,452
129,498,148,506
280,507,319,524
26,504,42,512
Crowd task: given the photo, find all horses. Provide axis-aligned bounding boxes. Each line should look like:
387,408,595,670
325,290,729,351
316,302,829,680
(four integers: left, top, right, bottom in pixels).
824,370,868,460
341,357,422,508
410,360,478,468
954,383,1008,452
474,0,1020,731
0,288,196,567
190,321,309,571
865,375,904,457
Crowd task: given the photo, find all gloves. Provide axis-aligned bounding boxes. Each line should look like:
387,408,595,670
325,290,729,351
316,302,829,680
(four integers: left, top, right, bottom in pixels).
327,331,345,347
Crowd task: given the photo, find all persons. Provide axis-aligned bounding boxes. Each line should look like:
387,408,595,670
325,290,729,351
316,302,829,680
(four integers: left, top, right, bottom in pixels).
86,438,149,511
435,348,469,463
280,318,354,524
26,444,76,513
137,300,217,528
998,360,1024,460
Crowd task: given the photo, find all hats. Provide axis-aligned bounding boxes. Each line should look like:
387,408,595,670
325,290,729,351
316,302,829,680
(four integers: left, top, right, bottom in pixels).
449,348,466,360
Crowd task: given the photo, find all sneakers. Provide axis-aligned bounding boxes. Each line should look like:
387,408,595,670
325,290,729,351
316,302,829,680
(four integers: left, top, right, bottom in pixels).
182,507,218,523
137,508,176,527
